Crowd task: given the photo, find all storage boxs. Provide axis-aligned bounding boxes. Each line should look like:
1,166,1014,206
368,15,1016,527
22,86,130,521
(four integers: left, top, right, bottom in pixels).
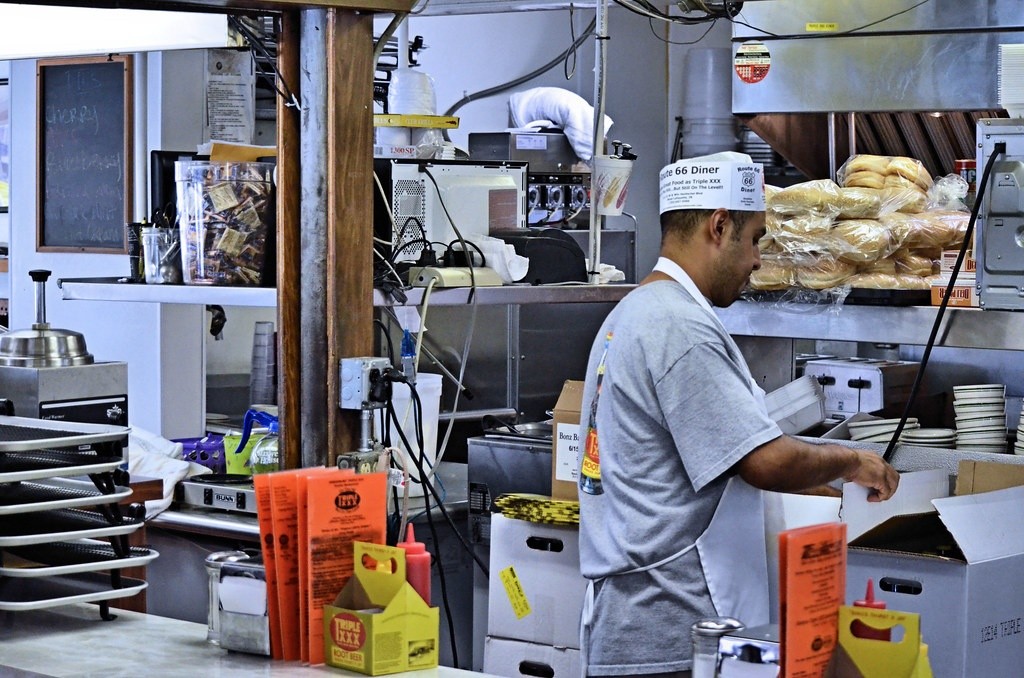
483,381,1024,678
323,540,439,676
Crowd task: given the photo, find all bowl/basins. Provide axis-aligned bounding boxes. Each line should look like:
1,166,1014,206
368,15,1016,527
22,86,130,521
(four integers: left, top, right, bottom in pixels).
846,415,920,450
997,43,1024,118
952,383,1024,455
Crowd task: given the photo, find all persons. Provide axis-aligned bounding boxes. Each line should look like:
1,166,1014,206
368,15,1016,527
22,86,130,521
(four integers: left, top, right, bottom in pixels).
579,152,900,677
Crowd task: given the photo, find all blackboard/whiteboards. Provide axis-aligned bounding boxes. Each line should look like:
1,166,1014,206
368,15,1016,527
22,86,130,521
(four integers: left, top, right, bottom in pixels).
35,54,134,254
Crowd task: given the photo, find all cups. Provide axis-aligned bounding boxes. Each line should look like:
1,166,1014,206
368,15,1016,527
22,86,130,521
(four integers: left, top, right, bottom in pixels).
592,153,633,217
250,322,274,405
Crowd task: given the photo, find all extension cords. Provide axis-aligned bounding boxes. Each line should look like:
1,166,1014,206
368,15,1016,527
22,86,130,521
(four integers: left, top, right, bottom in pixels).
409,267,504,287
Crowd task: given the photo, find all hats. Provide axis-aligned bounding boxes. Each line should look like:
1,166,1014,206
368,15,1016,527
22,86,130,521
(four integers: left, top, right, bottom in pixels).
659,151,767,215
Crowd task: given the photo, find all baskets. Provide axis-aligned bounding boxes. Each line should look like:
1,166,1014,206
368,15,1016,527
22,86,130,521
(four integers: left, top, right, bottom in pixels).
170,436,225,474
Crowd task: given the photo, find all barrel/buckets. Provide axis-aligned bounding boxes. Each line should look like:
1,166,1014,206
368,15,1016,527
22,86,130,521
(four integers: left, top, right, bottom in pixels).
390,371,442,497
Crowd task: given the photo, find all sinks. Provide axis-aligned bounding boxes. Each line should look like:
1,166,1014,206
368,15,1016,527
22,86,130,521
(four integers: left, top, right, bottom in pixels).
781,416,845,444
825,414,1024,476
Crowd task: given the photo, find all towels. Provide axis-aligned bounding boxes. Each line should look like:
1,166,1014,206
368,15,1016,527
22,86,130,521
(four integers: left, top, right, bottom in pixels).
507,86,615,161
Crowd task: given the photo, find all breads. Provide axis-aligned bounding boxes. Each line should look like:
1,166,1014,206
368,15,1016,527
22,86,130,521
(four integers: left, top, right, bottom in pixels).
744,156,975,291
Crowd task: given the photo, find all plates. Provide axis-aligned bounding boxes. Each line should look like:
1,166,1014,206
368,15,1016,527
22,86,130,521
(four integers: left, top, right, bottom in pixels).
898,427,956,448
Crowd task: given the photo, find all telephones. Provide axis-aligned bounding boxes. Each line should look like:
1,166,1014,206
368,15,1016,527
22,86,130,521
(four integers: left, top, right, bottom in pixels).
489,223,590,283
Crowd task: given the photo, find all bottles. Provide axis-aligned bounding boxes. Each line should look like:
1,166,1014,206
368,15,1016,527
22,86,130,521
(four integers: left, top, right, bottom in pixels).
205,549,251,645
691,615,745,678
397,523,431,608
845,580,889,677
